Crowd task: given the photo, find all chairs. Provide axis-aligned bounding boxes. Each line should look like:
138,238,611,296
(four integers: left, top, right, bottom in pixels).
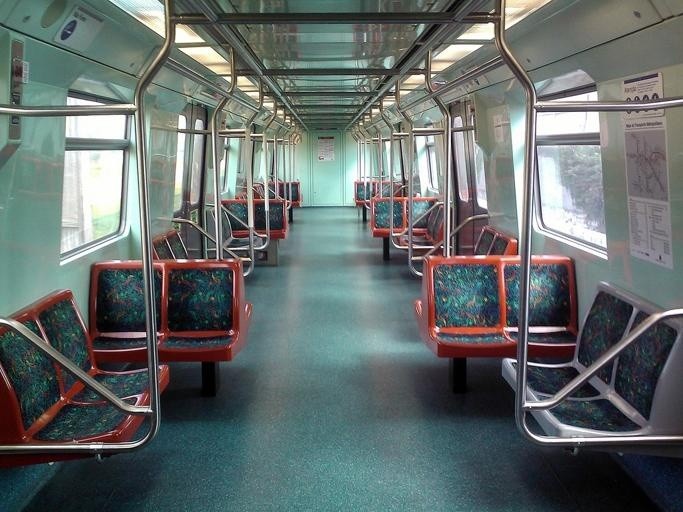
356,179,438,265
221,183,303,266
1,285,173,468
83,227,256,397
499,279,680,458
391,203,578,394
